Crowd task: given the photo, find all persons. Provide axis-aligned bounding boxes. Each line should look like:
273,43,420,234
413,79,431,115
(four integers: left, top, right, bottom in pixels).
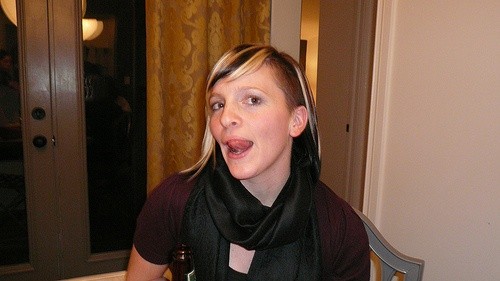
125,43,371,281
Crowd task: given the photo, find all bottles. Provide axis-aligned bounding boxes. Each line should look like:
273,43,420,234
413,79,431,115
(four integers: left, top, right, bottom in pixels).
172,240,197,280
